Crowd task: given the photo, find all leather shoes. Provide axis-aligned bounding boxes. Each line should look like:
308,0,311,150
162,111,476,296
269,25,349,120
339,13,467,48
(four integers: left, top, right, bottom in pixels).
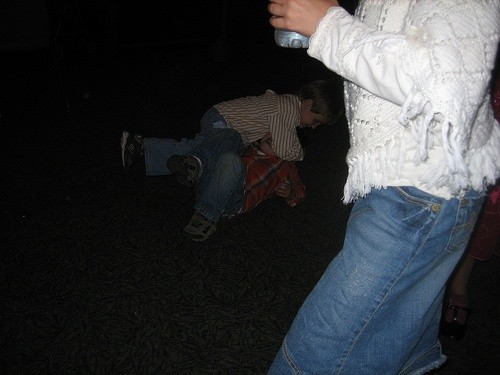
441,296,477,340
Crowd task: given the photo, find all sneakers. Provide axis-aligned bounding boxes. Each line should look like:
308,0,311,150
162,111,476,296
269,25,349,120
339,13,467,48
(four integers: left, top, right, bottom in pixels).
182,209,217,244
118,131,142,174
166,152,200,188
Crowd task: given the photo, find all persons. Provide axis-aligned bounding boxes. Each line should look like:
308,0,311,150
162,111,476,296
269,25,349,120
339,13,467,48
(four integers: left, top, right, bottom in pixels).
120,80,344,179
164,131,310,243
269,0,500,375
